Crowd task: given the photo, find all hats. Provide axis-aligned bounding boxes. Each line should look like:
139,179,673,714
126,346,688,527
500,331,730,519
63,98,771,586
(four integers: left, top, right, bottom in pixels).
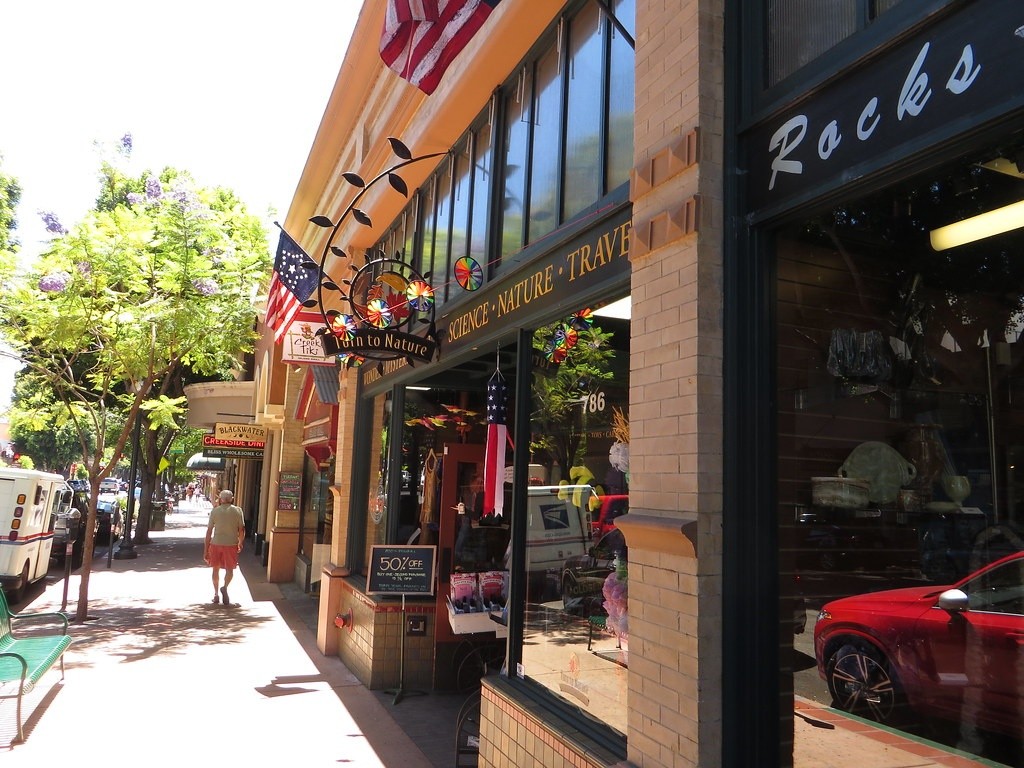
221,490,232,500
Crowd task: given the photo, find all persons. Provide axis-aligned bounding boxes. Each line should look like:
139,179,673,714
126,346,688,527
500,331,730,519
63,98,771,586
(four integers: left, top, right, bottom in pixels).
186,485,201,500
454,462,502,571
204,490,245,604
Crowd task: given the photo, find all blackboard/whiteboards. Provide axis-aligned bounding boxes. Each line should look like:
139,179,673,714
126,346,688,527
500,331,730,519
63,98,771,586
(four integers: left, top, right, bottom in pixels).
365,544,437,597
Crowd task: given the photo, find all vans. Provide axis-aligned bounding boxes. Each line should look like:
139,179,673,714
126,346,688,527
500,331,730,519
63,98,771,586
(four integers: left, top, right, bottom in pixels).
0,465,80,604
524,484,602,577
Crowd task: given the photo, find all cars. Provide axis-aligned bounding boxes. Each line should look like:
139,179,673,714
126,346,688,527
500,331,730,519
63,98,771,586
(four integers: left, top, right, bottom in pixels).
814,549,1024,742
48,477,168,567
590,495,631,542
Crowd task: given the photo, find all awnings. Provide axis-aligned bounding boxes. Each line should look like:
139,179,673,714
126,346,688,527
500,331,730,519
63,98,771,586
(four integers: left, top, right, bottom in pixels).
186,453,225,471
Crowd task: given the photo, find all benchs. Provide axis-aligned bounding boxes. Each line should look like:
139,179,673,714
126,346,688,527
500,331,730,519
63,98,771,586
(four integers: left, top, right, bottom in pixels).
0,581,71,743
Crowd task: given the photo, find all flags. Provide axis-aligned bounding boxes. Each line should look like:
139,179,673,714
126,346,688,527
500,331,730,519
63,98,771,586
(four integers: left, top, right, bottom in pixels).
265,229,327,345
379,0,501,96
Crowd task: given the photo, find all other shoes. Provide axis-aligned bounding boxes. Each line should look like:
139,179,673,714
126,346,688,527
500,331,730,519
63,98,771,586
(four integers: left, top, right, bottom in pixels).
212,597,219,603
221,587,229,605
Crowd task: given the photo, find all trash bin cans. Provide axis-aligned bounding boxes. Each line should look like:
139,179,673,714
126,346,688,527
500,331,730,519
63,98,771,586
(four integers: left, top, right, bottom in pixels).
172,491,179,506
149,501,166,531
181,489,186,499
